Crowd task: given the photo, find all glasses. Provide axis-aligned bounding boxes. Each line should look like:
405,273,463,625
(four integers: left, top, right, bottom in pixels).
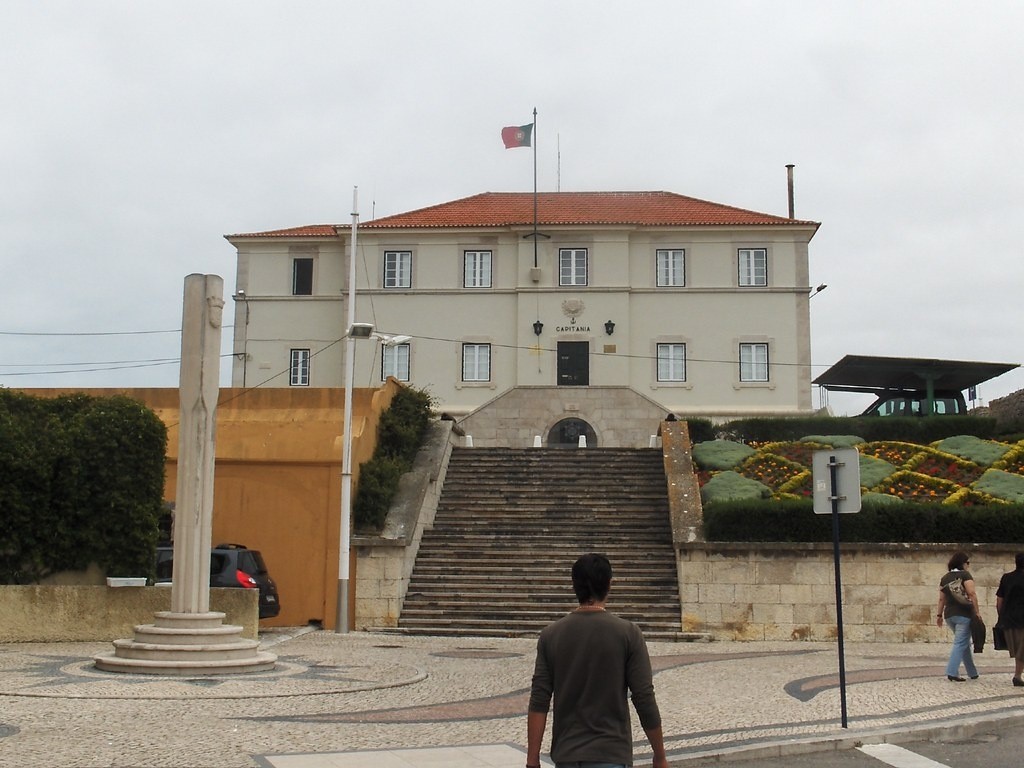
965,562,970,565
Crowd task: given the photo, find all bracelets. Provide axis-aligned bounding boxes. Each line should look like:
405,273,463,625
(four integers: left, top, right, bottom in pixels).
937,615,942,617
526,764,540,768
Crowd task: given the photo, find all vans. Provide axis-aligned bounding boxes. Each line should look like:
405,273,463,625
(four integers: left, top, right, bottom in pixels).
852,388,968,417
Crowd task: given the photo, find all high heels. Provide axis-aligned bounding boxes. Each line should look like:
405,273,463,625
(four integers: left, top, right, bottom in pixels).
948,674,966,682
971,675,979,679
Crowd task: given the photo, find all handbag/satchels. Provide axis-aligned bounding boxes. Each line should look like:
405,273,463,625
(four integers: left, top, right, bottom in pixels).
947,570,974,605
992,622,1009,650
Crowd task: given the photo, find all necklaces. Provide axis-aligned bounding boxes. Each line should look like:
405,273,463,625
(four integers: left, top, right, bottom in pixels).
576,606,604,610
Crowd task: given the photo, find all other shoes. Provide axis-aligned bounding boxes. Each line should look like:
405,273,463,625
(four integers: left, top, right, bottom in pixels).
1013,677,1024,686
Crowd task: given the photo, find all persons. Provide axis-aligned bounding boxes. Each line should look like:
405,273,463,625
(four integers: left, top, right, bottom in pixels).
996,553,1024,687
937,552,982,682
526,553,668,768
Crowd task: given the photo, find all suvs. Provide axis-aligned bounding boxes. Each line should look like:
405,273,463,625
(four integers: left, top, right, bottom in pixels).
155,543,280,619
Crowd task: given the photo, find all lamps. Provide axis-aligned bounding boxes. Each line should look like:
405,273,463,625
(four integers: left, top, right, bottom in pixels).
238,291,249,324
533,321,543,336
808,284,827,298
604,320,615,335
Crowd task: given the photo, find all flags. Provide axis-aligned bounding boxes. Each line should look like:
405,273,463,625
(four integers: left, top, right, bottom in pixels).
502,123,533,149
